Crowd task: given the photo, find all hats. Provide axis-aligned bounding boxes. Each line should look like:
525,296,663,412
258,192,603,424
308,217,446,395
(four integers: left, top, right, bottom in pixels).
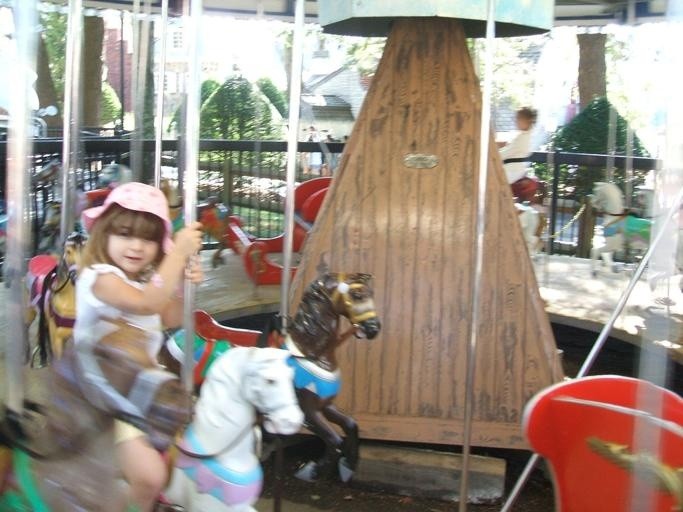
81,181,176,256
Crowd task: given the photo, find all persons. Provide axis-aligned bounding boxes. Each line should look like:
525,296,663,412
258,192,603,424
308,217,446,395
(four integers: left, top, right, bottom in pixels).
70,183,203,510
498,106,541,184
